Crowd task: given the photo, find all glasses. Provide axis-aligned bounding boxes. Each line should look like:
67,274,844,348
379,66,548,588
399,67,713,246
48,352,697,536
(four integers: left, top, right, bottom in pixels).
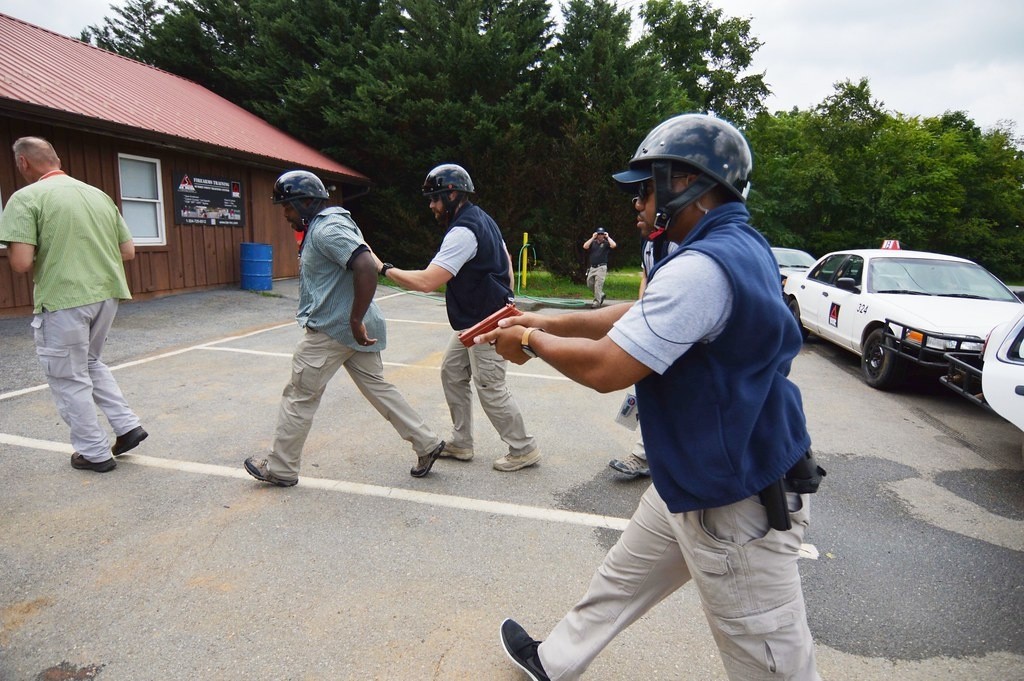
637,173,688,201
430,195,440,202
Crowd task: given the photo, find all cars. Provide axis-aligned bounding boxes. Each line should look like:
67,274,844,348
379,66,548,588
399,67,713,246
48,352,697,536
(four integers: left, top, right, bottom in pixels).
939,305,1024,435
770,246,817,272
782,240,1024,391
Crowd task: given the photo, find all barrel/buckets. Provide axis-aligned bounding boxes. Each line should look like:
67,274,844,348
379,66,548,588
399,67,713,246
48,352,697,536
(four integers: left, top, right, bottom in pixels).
239,243,273,292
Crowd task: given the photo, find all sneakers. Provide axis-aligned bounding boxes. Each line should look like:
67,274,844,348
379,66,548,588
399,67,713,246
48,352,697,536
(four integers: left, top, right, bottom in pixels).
244,457,298,487
440,440,474,460
494,444,541,471
500,618,551,681
410,441,446,477
609,453,651,476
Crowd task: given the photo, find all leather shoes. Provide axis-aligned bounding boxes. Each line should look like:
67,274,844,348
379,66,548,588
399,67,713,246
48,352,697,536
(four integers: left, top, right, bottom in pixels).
71,452,116,472
111,426,148,456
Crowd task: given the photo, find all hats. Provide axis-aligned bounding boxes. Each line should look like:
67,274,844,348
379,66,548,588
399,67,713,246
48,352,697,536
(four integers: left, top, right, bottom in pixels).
612,161,701,193
597,227,606,234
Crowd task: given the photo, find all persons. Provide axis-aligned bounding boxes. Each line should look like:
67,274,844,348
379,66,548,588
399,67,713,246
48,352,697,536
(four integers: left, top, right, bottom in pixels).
0,137,149,473
365,164,542,471
628,398,635,405
583,228,616,308
244,169,445,486
609,238,678,477
474,114,825,681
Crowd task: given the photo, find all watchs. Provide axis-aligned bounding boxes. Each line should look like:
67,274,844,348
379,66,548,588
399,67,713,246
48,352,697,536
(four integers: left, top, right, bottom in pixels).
380,263,393,276
521,327,546,359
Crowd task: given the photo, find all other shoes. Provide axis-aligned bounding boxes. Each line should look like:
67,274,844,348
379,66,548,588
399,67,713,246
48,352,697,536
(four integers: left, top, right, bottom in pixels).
591,301,601,308
600,294,606,304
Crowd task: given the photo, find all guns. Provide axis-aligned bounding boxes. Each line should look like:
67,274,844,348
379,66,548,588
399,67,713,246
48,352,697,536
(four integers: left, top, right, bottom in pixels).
758,476,792,532
459,301,521,350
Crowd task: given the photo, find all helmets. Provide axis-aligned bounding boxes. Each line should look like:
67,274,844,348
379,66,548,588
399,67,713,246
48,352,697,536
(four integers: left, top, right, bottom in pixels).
630,114,753,203
273,170,330,204
422,164,476,195
